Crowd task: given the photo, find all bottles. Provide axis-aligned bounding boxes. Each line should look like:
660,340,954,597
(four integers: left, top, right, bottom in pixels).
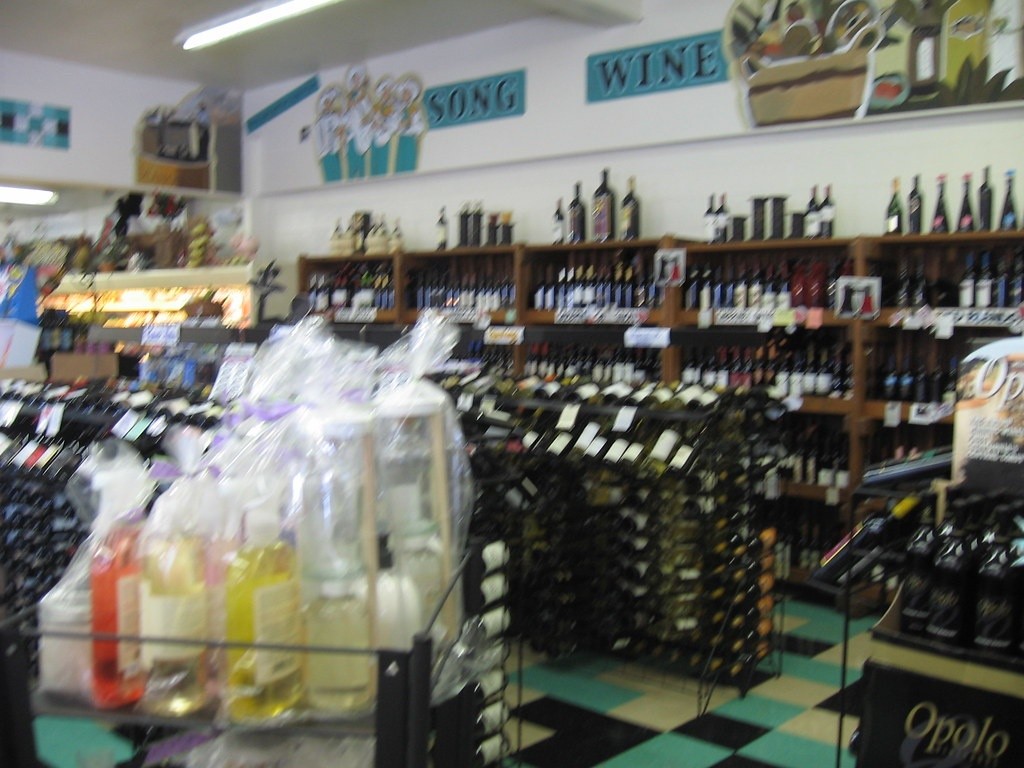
0,254,1024,768
908,175,923,234
802,185,836,237
999,170,1018,231
704,191,729,244
930,174,950,233
550,195,565,244
885,178,903,236
567,182,585,244
977,165,994,232
435,205,448,250
620,175,642,240
589,166,615,245
326,208,407,253
186,99,210,162
956,173,974,233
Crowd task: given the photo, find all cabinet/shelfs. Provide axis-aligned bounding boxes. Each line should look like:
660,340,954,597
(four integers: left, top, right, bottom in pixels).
300,231,1024,602
